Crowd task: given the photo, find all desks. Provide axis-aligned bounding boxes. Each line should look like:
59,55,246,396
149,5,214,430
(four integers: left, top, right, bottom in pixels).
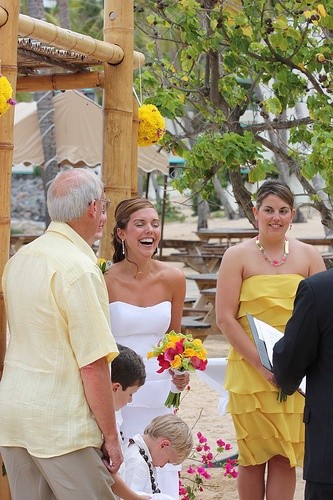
186,274,220,335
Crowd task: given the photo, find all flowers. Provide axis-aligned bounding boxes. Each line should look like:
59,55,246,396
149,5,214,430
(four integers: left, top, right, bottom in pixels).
146,330,210,410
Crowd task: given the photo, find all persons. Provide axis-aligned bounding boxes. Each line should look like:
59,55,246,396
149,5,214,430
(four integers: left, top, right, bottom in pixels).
215,181,327,500
0,168,192,500
273,270,333,500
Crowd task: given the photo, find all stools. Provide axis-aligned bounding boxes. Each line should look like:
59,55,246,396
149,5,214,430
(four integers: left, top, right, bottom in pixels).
178,297,211,343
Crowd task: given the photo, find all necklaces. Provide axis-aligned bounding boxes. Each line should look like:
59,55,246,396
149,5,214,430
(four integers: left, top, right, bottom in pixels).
128,439,161,494
256,235,289,267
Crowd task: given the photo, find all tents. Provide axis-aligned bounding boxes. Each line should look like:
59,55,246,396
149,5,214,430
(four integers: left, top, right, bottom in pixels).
13,90,169,260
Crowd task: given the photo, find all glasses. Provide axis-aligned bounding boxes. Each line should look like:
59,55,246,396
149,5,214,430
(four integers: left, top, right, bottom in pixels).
95,199,111,212
